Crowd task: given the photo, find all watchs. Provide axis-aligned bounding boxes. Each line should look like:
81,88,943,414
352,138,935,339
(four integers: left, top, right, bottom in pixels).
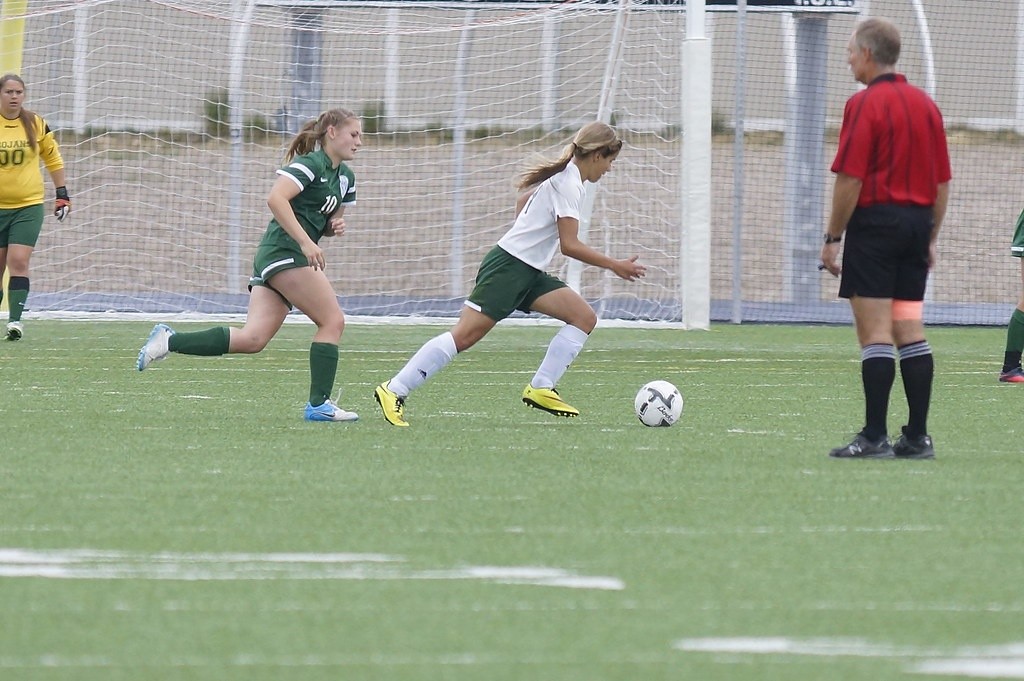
824,234,841,244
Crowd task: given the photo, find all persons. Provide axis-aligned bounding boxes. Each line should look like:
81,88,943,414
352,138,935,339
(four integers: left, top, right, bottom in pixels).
821,19,952,459
999,208,1024,383
373,121,647,426
136,108,362,422
0,75,72,342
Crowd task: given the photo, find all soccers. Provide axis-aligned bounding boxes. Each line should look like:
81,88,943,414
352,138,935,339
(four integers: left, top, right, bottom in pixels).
633,379,685,428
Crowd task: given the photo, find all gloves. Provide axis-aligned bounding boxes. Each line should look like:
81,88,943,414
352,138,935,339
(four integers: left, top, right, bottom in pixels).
54,196,71,221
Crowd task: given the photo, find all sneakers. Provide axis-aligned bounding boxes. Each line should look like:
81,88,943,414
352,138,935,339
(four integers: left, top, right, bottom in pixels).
5,321,24,342
374,380,410,427
1000,367,1024,382
304,388,358,422
829,426,934,459
136,324,175,371
522,383,579,417
